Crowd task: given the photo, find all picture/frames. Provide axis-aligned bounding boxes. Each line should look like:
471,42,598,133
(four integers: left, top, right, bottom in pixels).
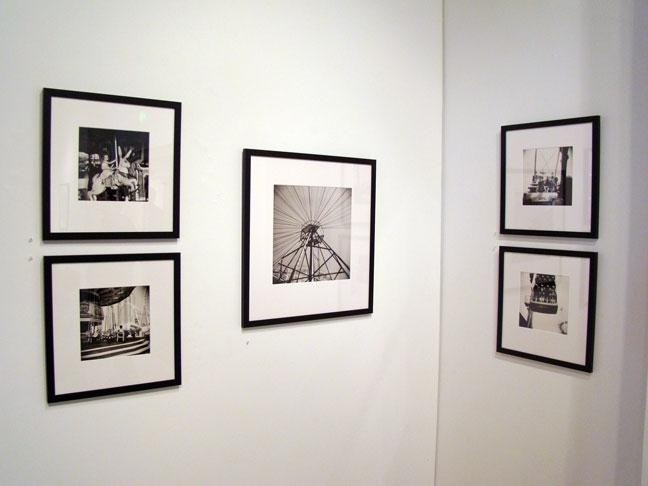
499,115,601,239
241,148,377,328
43,252,182,403
496,245,598,374
41,87,181,241
